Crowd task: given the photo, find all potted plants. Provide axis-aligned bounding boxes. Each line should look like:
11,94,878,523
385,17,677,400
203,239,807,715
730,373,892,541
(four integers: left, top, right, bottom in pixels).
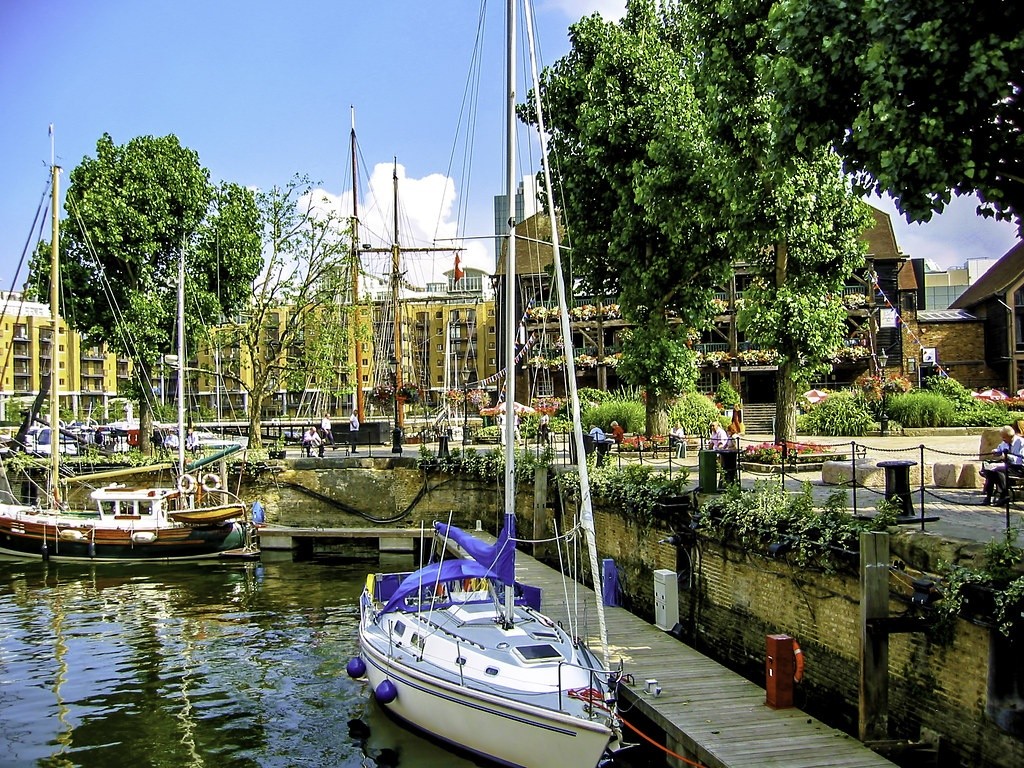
268,432,286,459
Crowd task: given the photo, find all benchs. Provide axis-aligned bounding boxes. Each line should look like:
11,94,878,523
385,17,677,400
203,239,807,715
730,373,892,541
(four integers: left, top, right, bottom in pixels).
300,443,353,458
652,441,710,459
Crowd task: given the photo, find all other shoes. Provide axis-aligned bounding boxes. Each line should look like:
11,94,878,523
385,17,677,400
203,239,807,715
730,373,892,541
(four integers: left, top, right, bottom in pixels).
318,454,325,457
307,454,316,457
995,496,1011,507
981,497,991,505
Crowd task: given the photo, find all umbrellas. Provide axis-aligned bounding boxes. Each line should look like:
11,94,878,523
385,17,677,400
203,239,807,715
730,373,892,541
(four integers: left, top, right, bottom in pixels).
484,400,535,415
978,389,1010,401
801,389,827,403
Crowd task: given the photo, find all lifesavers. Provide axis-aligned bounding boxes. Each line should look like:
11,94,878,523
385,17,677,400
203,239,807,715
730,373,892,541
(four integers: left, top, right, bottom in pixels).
780,635,806,686
201,473,221,492
177,473,197,494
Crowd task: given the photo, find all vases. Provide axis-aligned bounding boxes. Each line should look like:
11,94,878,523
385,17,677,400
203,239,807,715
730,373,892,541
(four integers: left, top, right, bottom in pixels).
528,315,622,324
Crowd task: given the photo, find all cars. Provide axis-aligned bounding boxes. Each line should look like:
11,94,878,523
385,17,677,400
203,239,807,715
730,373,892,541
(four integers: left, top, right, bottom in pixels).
22,426,80,456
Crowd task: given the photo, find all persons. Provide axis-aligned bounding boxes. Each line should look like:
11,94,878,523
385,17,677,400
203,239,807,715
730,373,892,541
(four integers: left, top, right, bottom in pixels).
163,428,200,456
497,410,506,447
669,421,686,459
589,425,605,441
320,412,337,451
299,427,324,457
708,421,739,449
540,410,550,446
610,420,623,444
349,410,360,454
514,409,524,446
982,419,1024,508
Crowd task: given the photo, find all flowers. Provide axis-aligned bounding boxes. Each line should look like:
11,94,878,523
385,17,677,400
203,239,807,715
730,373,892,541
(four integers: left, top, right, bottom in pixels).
529,353,622,371
527,304,622,319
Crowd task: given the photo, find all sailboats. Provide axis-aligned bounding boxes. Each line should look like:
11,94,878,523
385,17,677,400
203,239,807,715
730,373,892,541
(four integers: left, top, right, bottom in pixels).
352,0,637,768
2,123,258,564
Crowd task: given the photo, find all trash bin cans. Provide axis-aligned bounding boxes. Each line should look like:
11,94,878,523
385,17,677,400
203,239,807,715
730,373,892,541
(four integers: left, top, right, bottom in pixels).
877,460,918,516
699,450,716,493
715,450,738,488
571,430,593,466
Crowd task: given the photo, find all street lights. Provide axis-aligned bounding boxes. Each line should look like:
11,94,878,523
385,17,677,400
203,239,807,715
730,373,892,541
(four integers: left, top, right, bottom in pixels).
387,354,403,454
461,367,474,445
875,348,891,435
